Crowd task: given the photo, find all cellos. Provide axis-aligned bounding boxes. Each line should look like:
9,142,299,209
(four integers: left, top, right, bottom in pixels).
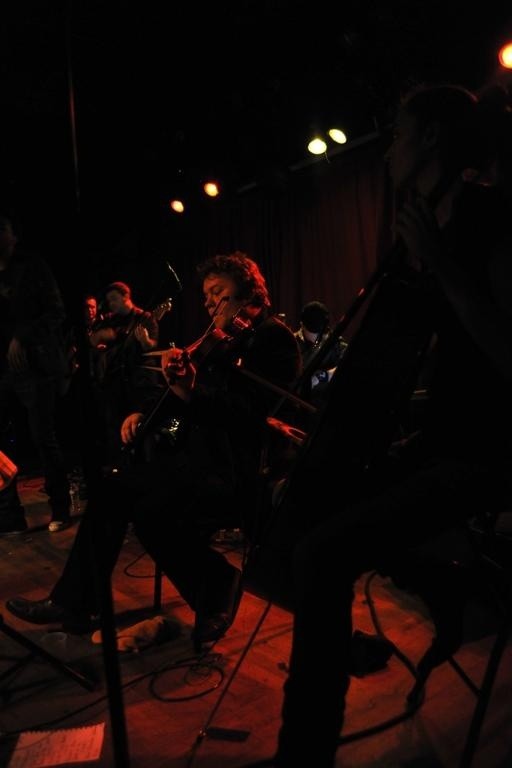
263,165,464,582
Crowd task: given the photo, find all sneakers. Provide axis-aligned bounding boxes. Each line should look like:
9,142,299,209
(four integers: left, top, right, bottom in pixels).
48,518,69,533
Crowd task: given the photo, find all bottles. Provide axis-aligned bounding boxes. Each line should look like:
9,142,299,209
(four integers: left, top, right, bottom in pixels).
68,483,81,513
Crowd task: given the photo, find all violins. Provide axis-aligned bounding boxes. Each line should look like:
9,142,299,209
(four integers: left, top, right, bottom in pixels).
164,302,264,385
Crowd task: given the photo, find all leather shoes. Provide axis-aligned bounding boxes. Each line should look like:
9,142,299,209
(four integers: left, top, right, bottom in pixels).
5,595,97,633
191,567,243,643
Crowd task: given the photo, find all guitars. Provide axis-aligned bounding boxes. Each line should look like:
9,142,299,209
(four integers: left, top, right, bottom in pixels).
96,299,173,392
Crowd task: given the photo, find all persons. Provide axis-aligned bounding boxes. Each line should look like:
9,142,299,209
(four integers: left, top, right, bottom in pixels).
237,83,512,767
